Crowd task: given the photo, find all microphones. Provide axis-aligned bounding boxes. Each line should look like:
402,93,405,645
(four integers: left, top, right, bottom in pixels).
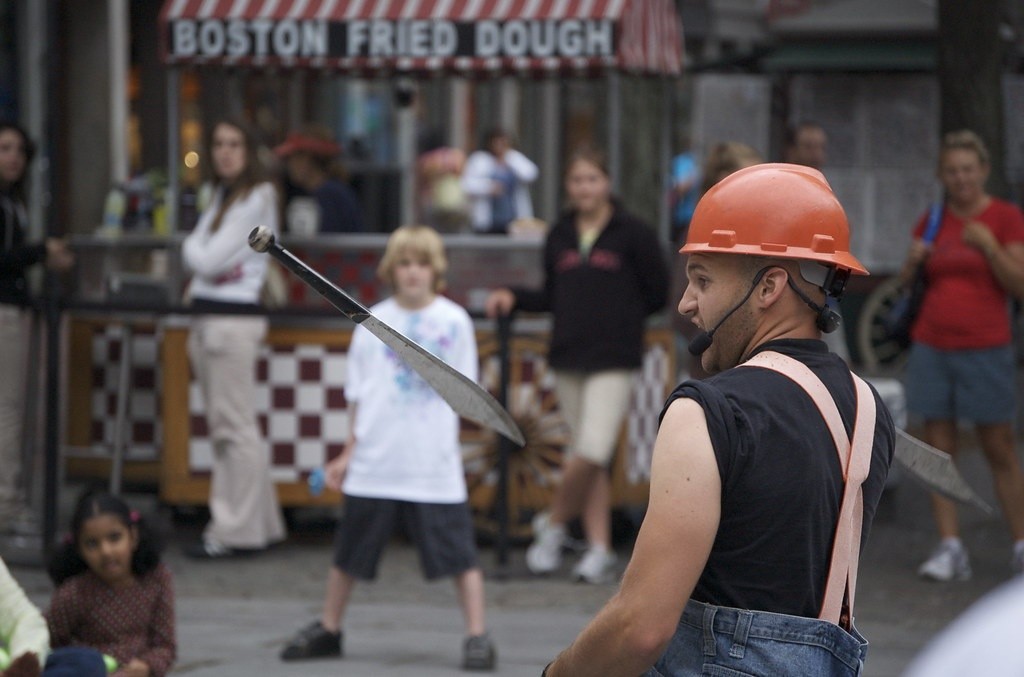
688,328,715,356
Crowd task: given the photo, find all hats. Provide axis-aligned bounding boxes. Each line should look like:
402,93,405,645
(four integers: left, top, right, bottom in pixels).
275,129,338,156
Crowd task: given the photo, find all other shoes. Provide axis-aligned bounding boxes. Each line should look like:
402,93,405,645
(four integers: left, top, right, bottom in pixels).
574,543,618,584
184,540,278,561
279,619,343,660
0,507,45,539
918,543,970,582
525,512,568,574
464,633,498,670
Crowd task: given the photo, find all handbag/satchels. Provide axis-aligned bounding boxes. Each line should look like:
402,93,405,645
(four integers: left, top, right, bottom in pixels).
882,268,931,349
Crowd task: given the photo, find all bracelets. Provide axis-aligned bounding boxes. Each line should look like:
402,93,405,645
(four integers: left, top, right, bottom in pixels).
541,662,550,677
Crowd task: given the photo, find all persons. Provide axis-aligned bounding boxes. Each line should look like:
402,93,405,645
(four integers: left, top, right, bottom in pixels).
422,128,540,235
0,117,65,563
540,162,897,677
184,116,287,558
273,130,367,237
526,142,759,585
783,122,830,170
280,225,498,668
0,559,107,677
904,134,1024,582
43,494,176,677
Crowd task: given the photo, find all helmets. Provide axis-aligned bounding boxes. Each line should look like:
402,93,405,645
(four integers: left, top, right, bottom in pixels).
679,163,869,276
